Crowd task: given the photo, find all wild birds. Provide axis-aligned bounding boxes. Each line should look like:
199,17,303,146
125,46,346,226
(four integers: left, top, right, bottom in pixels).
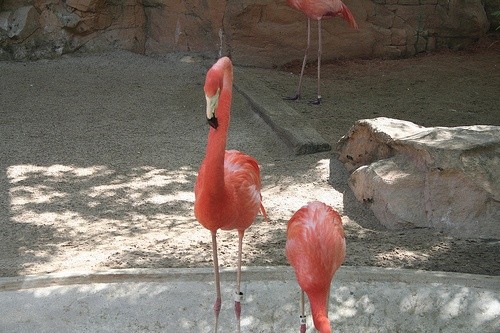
193,56,268,333
280,0,359,106
284,200,347,333
